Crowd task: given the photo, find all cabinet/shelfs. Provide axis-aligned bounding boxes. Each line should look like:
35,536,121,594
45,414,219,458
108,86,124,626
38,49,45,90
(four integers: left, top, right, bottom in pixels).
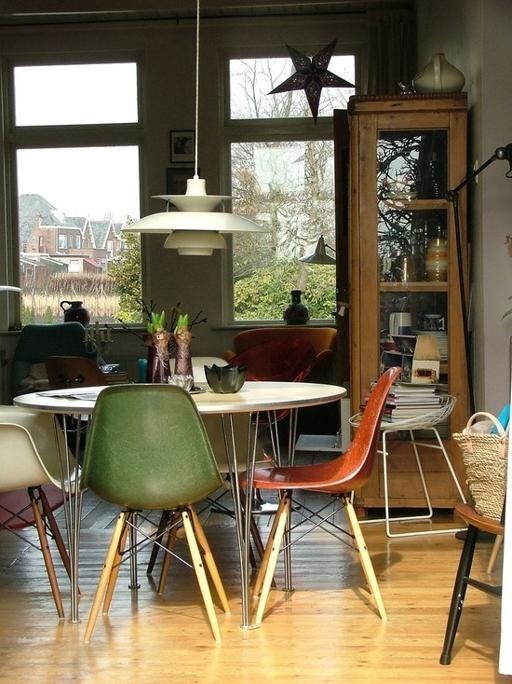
344,91,472,438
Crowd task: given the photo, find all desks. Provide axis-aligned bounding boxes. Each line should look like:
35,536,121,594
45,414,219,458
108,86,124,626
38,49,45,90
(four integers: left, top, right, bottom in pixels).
8,372,347,585
295,431,342,470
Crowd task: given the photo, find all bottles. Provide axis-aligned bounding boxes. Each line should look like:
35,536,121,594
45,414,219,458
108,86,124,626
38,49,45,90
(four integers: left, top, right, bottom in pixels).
424,237,446,282
411,53,466,91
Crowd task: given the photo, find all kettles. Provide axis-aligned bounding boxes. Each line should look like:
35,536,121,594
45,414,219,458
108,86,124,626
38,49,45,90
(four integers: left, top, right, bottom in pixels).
389,313,414,345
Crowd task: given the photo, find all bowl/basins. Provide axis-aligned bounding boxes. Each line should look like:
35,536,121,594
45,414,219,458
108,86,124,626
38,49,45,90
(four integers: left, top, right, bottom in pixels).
202,363,249,393
169,374,194,393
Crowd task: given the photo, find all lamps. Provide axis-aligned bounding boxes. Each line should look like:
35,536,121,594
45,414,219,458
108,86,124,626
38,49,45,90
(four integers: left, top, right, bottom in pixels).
123,0,257,260
440,140,511,424
298,232,341,266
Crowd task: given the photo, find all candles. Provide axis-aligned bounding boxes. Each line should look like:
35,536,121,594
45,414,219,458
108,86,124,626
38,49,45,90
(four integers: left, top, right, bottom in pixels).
83,321,113,341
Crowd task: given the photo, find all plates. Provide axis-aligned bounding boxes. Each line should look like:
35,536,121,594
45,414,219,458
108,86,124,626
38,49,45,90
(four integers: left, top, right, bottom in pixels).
416,330,449,361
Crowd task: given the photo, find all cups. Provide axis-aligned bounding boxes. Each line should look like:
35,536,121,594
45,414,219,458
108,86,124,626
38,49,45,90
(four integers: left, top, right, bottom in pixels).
423,313,445,331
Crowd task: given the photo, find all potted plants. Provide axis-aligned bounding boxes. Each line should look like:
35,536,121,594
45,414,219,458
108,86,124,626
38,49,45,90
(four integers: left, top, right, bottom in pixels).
116,293,208,389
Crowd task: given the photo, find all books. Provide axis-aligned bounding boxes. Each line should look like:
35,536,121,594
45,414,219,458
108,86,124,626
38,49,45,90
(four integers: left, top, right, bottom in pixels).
360,379,444,423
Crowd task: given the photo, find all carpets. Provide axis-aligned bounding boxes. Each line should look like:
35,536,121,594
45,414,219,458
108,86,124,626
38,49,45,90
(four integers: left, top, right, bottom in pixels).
0,477,73,531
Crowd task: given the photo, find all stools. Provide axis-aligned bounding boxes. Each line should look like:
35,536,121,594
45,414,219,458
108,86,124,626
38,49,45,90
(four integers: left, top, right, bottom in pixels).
440,502,503,666
345,392,478,537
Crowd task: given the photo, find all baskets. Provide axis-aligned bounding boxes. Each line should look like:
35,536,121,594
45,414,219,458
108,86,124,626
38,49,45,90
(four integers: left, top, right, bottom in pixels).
452,411,509,522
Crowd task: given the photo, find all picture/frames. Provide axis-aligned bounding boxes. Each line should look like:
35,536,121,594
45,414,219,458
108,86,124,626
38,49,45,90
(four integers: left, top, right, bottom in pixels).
167,130,196,164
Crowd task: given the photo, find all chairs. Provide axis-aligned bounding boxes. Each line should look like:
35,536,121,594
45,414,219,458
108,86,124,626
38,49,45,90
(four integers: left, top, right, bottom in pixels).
1,322,111,407
0,404,87,617
240,367,402,630
42,354,122,467
147,355,277,574
77,384,227,642
223,322,341,430
